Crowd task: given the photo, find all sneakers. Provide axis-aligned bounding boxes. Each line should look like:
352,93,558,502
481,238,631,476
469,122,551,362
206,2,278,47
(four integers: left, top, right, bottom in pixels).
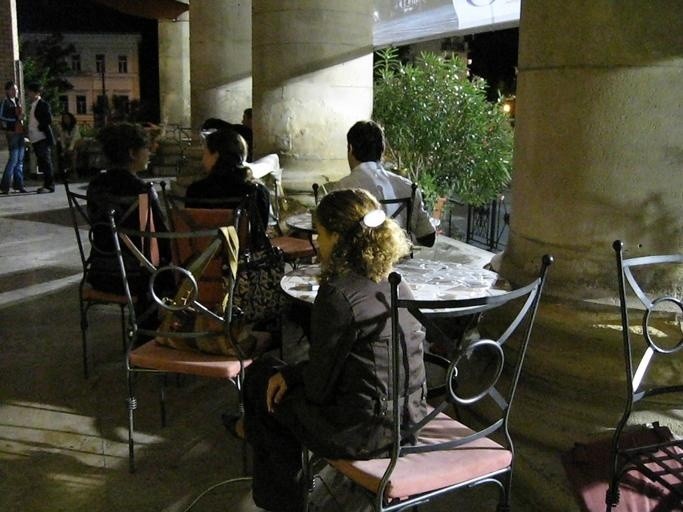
0,186,54,195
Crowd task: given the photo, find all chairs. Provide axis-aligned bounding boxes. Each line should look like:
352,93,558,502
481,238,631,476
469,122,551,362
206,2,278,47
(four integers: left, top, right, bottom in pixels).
243,153,313,262
159,181,258,309
312,183,417,258
107,209,263,477
606,241,683,511
65,178,173,382
300,255,554,512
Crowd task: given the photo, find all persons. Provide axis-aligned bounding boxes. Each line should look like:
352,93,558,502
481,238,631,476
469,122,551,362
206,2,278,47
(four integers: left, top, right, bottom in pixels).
184,128,294,356
27,82,56,194
319,120,436,248
220,188,429,512
202,108,251,163
0,81,28,195
87,121,172,350
60,112,83,181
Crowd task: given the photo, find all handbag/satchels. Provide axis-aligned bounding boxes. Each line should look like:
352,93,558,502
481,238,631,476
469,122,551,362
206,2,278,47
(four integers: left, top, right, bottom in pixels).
156,313,251,358
221,248,287,323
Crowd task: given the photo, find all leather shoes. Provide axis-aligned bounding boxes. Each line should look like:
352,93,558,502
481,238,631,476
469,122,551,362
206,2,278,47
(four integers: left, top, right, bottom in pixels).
220,412,244,439
